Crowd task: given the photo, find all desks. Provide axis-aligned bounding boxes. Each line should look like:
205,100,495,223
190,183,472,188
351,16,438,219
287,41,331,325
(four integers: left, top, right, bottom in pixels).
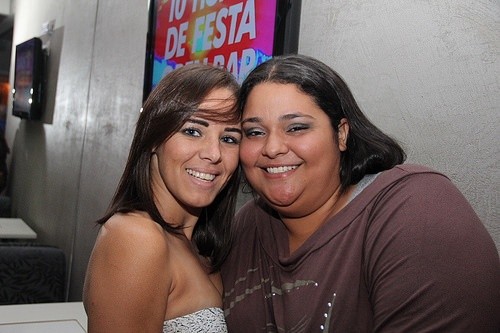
0,302,87,333
0,218,37,238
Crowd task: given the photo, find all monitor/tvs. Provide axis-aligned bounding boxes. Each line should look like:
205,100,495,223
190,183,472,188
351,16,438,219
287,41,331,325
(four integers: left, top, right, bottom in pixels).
142,0,302,110
13,37,45,118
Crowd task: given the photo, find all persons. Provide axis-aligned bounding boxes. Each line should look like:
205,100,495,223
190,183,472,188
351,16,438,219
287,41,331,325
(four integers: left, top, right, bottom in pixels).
81,62,242,332
222,55,500,333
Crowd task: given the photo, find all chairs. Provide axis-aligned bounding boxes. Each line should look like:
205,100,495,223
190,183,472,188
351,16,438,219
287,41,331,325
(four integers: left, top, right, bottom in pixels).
0,242,74,305
